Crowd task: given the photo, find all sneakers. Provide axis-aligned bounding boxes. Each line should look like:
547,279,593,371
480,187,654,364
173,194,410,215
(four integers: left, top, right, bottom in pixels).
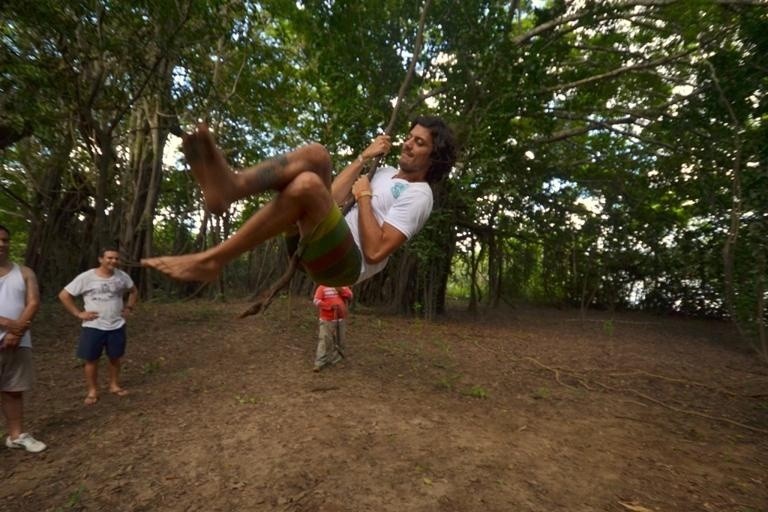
6,432,47,453
336,362,345,369
313,365,326,372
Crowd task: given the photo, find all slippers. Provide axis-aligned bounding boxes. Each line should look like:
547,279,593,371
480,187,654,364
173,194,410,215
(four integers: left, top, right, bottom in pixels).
85,396,99,406
108,387,128,397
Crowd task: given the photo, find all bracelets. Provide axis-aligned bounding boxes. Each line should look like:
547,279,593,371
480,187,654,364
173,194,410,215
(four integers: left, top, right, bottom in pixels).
357,153,366,165
126,306,133,313
356,191,372,201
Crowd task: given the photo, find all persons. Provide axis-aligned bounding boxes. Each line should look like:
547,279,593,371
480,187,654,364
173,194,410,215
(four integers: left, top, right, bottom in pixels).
139,116,457,287
1,226,47,453
312,284,354,372
58,246,138,406
